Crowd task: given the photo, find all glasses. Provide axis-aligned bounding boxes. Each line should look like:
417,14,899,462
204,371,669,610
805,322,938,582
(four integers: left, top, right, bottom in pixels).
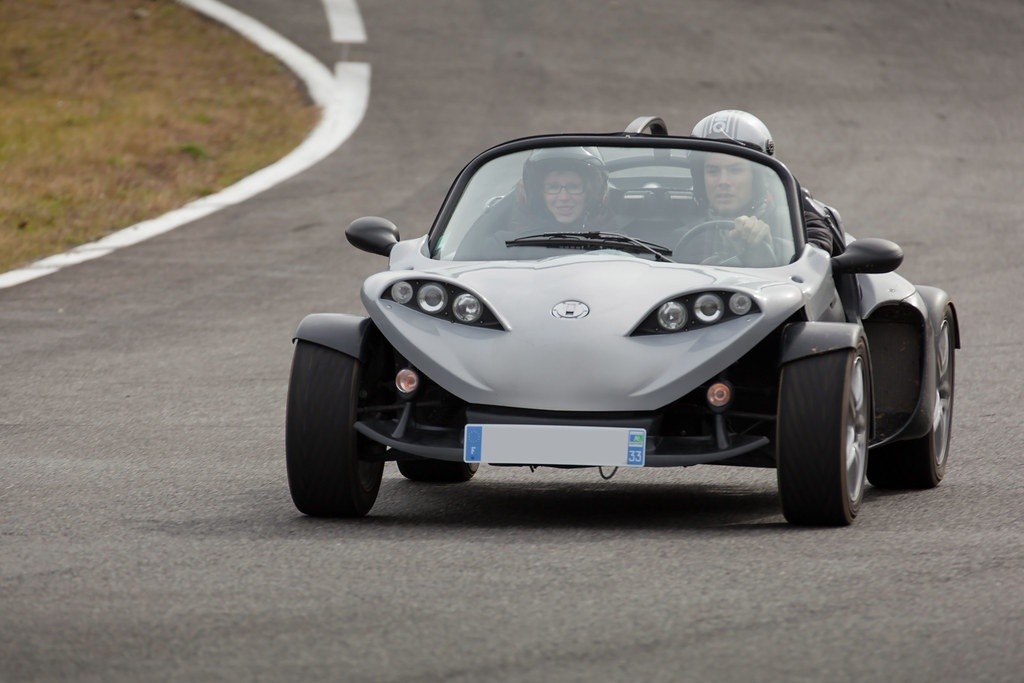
540,182,587,195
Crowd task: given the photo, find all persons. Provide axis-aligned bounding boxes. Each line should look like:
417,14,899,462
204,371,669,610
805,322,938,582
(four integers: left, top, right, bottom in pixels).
670,110,790,266
471,147,659,260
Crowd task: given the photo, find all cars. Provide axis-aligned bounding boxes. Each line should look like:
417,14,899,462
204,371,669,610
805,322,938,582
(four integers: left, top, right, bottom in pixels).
285,115,962,530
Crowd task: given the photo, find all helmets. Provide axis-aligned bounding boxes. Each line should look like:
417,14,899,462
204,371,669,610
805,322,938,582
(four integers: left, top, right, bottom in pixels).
523,144,609,213
688,108,778,208
